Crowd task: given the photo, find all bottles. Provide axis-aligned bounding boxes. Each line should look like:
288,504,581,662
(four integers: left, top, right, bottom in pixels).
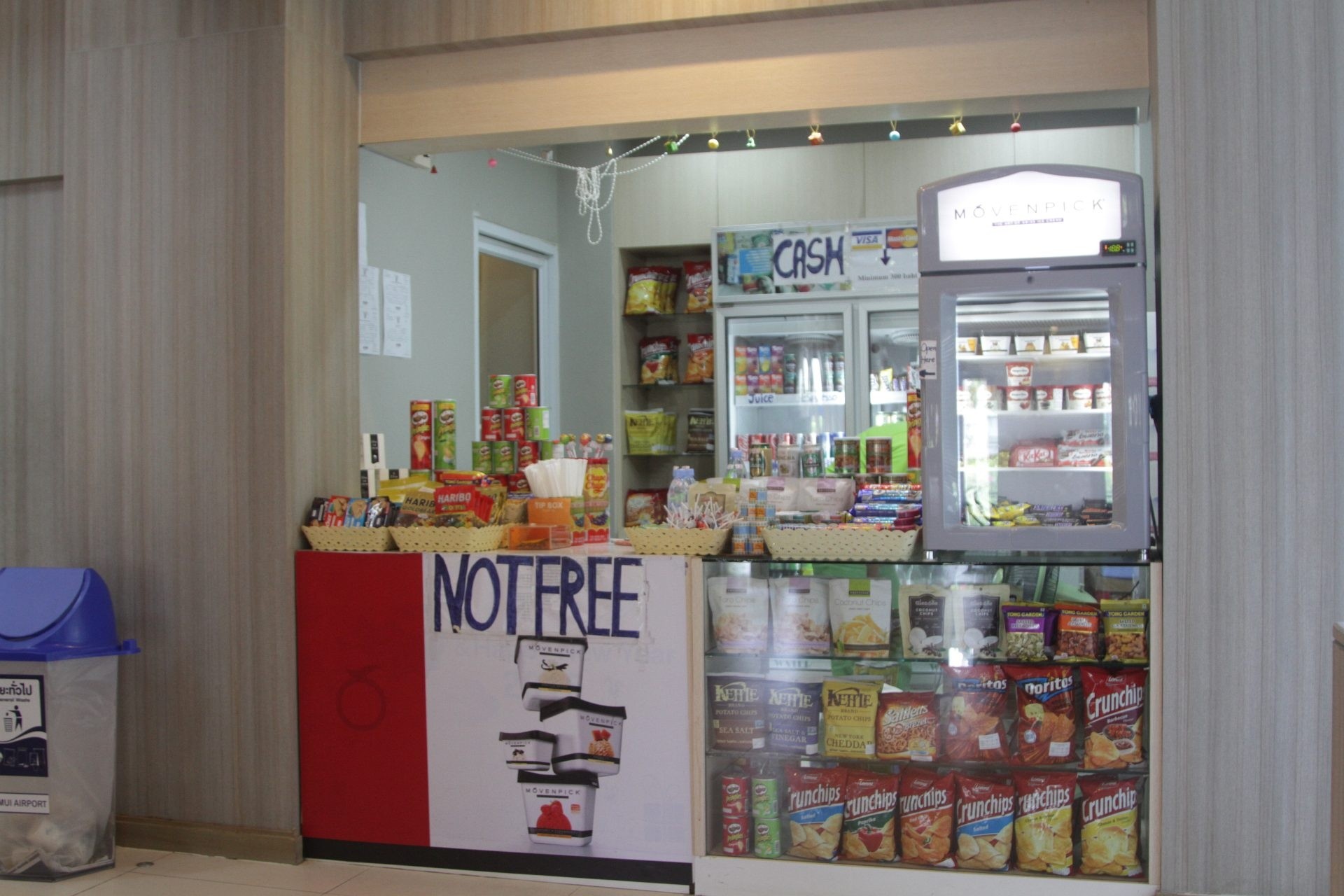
834,437,861,473
492,441,514,473
471,441,492,474
482,406,503,441
685,468,695,485
408,471,443,483
514,374,538,407
508,474,531,493
503,407,525,440
525,406,549,441
724,452,747,493
732,488,775,556
667,469,690,510
541,440,563,460
825,474,907,493
433,400,457,470
721,773,782,858
518,441,539,470
486,474,508,486
409,400,433,470
489,374,512,407
866,437,892,473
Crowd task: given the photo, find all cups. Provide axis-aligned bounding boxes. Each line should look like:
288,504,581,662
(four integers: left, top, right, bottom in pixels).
958,330,1111,355
995,361,1112,410
25,817,63,853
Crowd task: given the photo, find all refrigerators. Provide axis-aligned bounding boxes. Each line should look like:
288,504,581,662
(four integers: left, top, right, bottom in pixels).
916,164,1150,564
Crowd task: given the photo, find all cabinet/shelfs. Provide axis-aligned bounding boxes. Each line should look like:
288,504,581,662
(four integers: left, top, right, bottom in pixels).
619,311,715,458
689,551,1164,895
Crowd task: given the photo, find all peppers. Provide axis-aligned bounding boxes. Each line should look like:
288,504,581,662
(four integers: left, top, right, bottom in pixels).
858,823,884,852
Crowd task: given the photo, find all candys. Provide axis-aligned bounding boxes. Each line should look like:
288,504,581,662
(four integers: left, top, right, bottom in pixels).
560,433,612,458
663,493,743,529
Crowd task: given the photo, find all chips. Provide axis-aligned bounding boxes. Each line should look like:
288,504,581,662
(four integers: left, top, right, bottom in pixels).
1015,818,1139,875
901,815,951,862
843,817,896,860
1085,732,1128,769
955,821,1013,870
786,813,843,859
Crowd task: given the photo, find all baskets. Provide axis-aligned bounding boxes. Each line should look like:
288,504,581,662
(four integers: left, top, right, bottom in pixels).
389,526,502,553
302,526,394,552
502,524,524,546
626,527,732,556
760,527,920,563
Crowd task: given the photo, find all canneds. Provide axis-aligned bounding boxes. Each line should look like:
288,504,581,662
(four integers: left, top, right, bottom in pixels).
737,431,845,458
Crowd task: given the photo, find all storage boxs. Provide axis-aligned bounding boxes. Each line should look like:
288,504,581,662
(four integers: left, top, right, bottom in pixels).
508,526,572,550
529,498,587,546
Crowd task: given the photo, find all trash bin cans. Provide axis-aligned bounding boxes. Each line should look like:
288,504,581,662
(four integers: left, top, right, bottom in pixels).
0,567,142,883
